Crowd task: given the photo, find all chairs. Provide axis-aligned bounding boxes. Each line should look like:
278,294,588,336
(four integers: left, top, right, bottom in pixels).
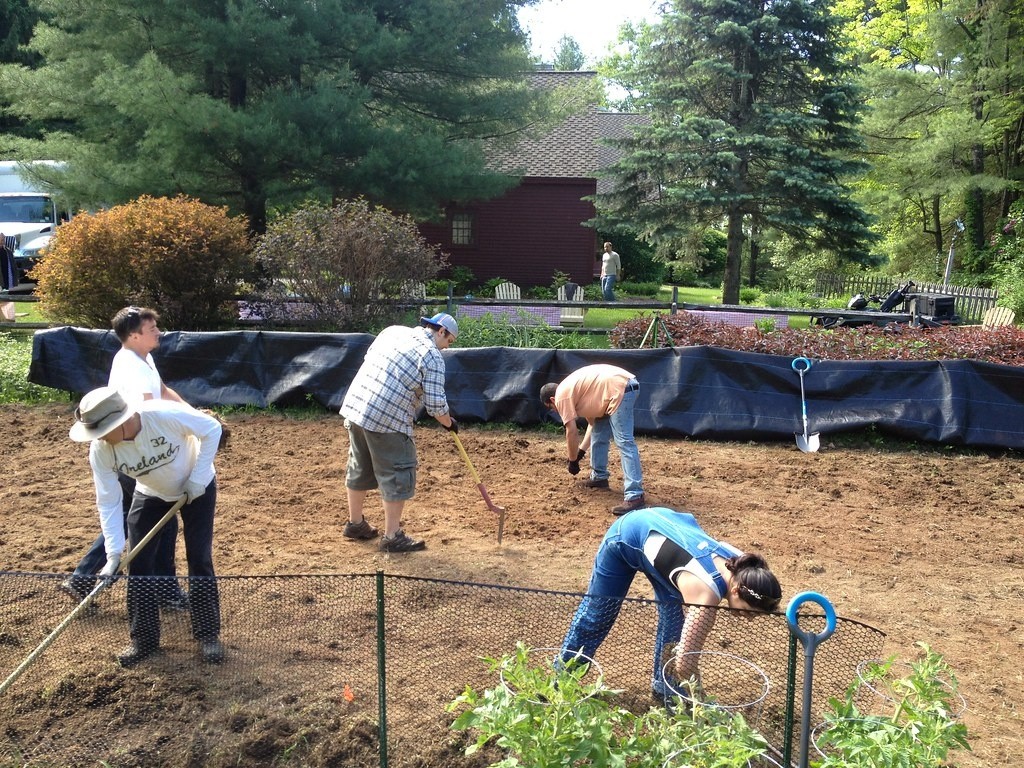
495,282,521,300
558,284,585,327
401,279,428,314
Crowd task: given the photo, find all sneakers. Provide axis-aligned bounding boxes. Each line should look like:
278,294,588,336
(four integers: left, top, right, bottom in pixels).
118,640,156,664
379,529,426,552
201,635,224,662
343,515,379,538
611,498,645,514
579,479,608,487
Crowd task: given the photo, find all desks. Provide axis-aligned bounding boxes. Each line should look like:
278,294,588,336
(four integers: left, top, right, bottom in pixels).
957,308,1016,330
456,304,588,326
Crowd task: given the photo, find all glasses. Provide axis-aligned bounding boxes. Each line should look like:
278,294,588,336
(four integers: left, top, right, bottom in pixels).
743,587,782,608
126,305,139,332
74,407,122,429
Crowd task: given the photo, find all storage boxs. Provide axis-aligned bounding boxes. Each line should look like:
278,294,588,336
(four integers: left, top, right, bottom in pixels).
906,292,957,319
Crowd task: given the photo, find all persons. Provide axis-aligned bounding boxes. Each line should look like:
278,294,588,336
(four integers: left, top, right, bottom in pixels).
550,507,783,724
540,363,647,515
599,241,622,300
62,306,197,613
68,386,223,666
339,312,459,553
0,232,19,290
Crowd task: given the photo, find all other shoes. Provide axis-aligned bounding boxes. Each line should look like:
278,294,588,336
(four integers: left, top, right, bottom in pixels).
62,580,100,611
161,591,191,611
651,690,676,706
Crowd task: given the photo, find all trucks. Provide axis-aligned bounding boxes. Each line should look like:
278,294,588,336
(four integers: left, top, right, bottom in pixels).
0,158,140,290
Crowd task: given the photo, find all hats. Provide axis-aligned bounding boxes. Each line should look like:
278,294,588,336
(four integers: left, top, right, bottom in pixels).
421,312,458,339
69,386,144,442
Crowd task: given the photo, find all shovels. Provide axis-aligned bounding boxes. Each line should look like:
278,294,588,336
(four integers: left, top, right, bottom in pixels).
449,430,504,546
792,358,820,453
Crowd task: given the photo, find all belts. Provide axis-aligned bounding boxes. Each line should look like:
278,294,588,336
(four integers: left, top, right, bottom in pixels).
625,385,639,393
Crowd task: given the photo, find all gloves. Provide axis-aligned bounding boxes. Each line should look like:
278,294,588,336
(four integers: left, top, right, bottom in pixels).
442,417,458,434
578,448,586,461
176,479,204,504
568,459,580,474
100,556,124,589
